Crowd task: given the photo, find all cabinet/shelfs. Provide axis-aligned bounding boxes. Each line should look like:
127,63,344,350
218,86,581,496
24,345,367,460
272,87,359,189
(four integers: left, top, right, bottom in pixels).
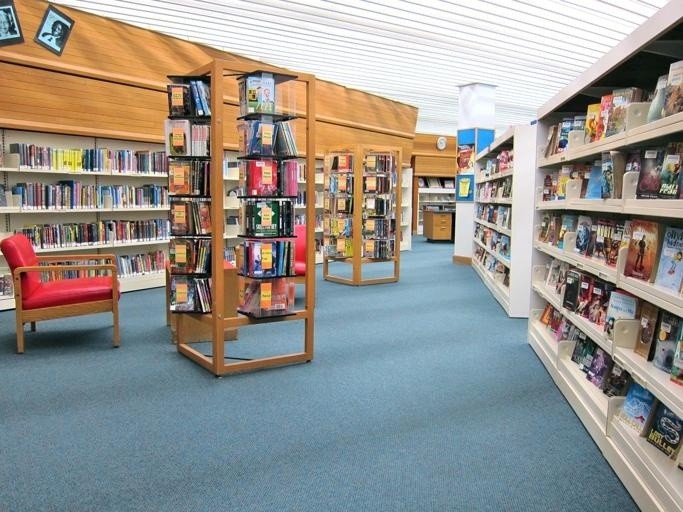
413,175,455,235
1,114,167,308
470,125,536,318
167,58,324,378
434,213,453,241
324,143,412,286
528,1,683,512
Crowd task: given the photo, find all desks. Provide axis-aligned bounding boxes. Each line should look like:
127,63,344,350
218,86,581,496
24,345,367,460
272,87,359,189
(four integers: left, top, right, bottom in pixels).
423,210,455,242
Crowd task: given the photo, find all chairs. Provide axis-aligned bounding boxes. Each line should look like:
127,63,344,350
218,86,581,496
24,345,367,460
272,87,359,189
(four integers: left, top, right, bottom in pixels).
3,233,120,354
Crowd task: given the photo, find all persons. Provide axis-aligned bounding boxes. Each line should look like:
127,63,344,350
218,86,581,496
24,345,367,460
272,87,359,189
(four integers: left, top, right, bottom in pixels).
0,9,16,38
42,20,65,49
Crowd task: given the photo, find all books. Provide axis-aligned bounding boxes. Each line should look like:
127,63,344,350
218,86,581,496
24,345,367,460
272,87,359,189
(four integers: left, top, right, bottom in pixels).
200,281,211,313
287,277,296,313
315,150,395,260
3,180,170,210
163,75,296,140
235,279,272,319
197,280,209,312
166,142,212,314
473,150,514,294
540,302,681,469
2,250,168,295
459,174,475,202
274,277,288,319
459,144,475,173
536,211,680,292
224,140,306,317
543,64,681,158
10,144,166,172
2,219,168,249
542,142,679,200
168,275,206,314
544,257,680,383
